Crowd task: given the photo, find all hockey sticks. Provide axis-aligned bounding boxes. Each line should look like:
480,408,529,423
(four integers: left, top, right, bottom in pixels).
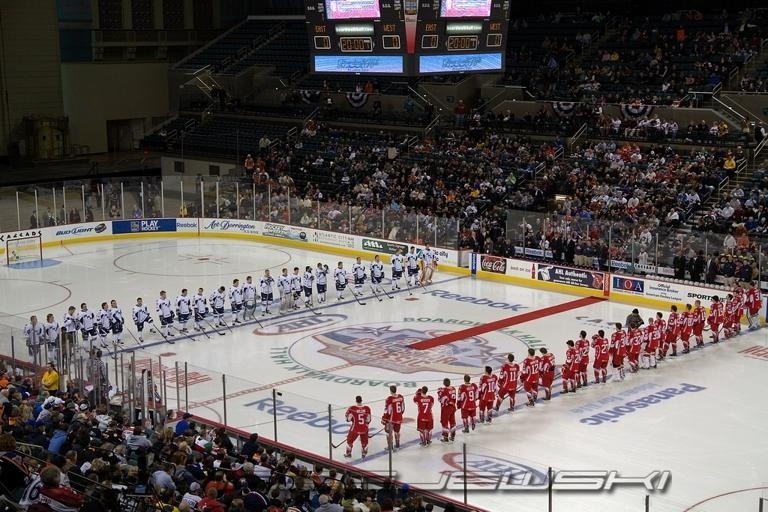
414,275,428,291
290,279,296,310
331,438,348,449
150,321,175,344
372,277,394,299
494,372,563,400
346,284,366,305
323,264,328,301
294,293,322,316
94,330,119,359
100,327,134,353
162,319,196,341
677,320,726,338
122,323,145,350
367,427,386,439
403,271,413,295
214,306,263,333
93,266,428,361
196,312,225,336
365,277,382,301
265,282,272,314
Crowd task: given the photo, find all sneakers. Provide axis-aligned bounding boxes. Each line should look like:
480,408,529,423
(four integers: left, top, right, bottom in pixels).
354,282,421,296
657,326,762,361
280,296,344,314
559,364,657,393
344,424,477,461
43,310,275,364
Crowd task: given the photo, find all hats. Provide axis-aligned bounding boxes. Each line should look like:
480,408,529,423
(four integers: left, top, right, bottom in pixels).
183,413,193,419
43,396,92,411
477,397,552,424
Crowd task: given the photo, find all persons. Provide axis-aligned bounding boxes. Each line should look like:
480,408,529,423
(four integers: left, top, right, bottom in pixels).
179,1,767,289
29,178,162,231
1,375,481,511
481,290,762,427
23,289,382,376
